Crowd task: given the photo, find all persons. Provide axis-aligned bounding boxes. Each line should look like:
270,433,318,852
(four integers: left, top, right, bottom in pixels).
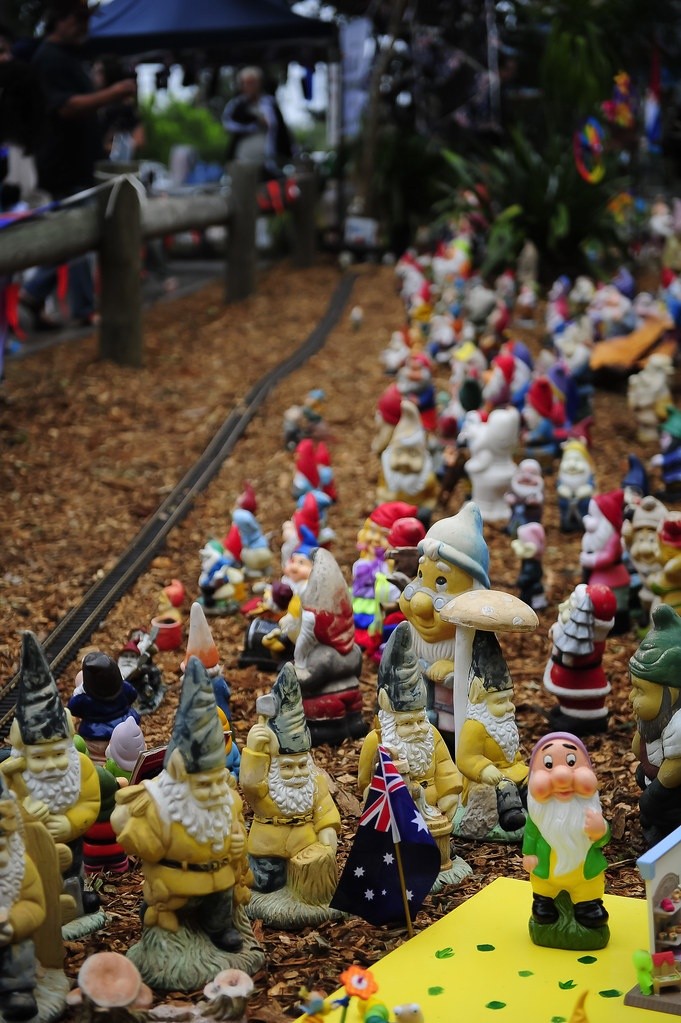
0,242,681,1023
222,66,295,253
0,0,174,344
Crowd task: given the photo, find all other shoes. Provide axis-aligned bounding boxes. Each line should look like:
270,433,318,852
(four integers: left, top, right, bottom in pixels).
70,300,102,328
10,298,66,334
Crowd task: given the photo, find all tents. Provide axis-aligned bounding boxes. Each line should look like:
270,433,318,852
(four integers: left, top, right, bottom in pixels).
69,0,342,245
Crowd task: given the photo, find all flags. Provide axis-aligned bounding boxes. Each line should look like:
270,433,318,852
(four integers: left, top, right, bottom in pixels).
329,745,442,927
